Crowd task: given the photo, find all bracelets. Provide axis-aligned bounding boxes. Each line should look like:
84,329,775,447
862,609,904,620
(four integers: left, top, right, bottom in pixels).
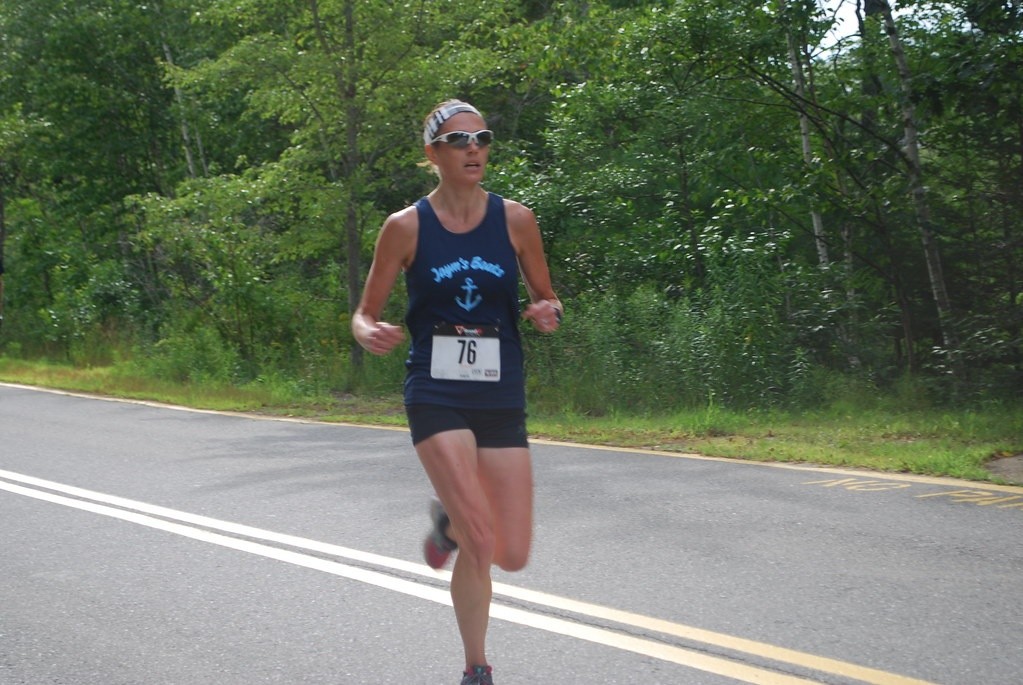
553,306,562,322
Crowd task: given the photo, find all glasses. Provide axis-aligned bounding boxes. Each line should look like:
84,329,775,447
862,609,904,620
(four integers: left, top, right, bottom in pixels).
429,127,496,149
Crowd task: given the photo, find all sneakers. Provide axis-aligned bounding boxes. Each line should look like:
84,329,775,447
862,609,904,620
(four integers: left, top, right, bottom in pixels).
424,497,458,569
460,666,494,685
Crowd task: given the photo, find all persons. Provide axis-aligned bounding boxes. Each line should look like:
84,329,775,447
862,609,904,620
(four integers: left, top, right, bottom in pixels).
352,98,564,684
